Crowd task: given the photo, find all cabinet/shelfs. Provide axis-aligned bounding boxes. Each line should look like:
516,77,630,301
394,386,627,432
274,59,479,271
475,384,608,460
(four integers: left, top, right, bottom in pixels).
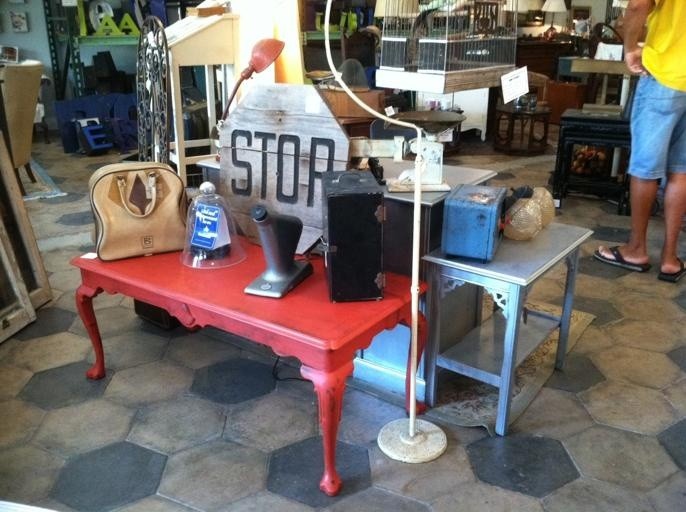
420,223,595,437
518,40,568,81
195,157,498,409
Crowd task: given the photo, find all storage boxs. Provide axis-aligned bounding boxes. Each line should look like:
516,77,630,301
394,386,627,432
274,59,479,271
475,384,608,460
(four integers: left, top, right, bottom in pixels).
441,183,507,261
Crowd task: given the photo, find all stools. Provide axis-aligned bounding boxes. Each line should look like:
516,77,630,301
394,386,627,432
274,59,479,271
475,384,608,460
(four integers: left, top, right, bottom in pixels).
79,124,115,156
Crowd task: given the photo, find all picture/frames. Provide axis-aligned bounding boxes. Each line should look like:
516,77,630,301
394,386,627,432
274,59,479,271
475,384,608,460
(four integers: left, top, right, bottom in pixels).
572,7,590,31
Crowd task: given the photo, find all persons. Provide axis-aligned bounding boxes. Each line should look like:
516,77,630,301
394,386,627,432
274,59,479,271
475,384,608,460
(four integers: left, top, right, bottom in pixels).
590,0,686,283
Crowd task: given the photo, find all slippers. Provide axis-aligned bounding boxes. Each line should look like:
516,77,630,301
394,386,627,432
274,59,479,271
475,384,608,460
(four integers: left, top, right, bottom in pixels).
593,245,653,273
658,256,686,283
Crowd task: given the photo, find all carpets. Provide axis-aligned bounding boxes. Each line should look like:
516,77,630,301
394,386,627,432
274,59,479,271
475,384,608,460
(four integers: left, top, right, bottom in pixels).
17,155,68,201
425,289,597,438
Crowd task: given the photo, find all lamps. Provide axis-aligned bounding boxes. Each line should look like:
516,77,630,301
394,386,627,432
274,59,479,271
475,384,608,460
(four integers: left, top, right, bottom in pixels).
541,1,567,42
224,38,283,117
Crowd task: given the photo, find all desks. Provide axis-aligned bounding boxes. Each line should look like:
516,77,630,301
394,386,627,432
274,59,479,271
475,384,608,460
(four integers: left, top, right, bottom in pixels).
493,99,553,157
393,111,467,157
69,242,429,497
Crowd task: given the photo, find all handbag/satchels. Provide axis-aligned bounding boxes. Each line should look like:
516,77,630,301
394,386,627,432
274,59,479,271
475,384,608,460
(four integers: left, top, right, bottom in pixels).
86,159,194,262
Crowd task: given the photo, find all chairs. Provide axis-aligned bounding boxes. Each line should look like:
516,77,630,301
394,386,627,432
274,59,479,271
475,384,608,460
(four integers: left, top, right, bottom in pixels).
0,59,43,197
553,78,637,214
